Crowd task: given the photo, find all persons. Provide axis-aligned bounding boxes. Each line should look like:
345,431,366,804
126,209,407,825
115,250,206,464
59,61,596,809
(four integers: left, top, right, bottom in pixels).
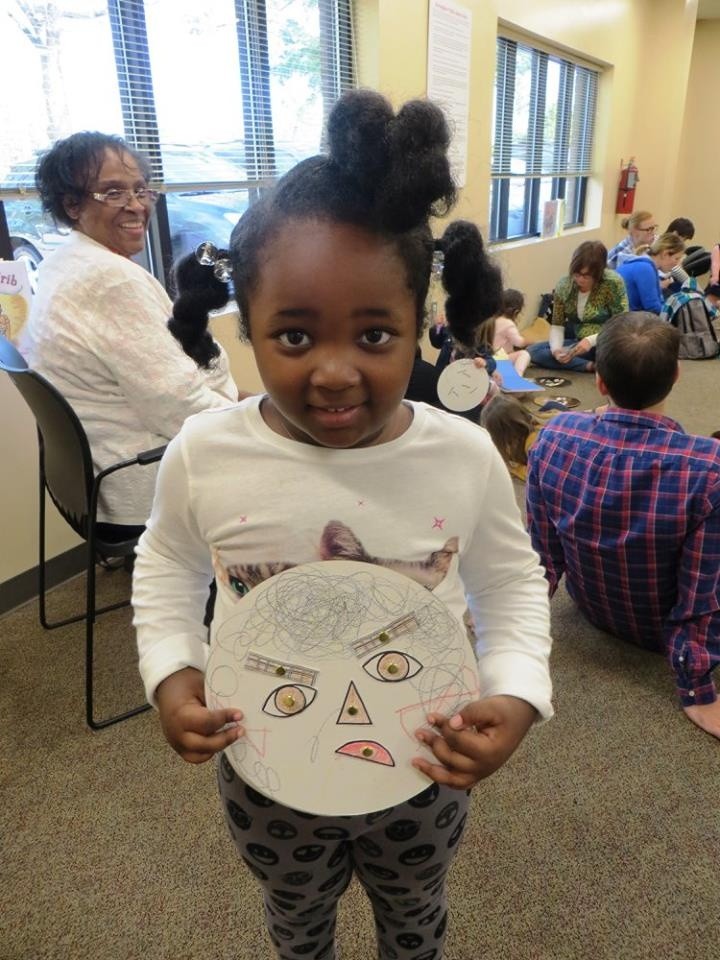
523,311,720,741
130,89,554,960
404,211,720,484
16,132,239,536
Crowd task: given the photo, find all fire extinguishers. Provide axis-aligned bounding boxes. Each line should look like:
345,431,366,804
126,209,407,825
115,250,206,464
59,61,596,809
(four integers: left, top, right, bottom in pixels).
616,157,638,214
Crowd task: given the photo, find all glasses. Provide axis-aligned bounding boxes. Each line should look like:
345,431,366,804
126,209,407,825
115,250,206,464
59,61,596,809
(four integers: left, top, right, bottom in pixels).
574,270,593,280
637,225,658,233
84,189,159,208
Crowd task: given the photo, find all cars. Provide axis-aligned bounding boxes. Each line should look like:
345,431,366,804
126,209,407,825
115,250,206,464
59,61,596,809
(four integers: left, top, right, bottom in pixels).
2,139,299,301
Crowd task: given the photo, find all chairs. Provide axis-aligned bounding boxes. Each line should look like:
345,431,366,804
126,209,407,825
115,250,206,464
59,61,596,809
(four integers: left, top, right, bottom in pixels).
0,335,167,730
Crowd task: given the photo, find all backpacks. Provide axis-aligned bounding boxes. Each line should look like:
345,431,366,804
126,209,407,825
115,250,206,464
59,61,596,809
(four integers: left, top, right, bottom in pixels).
659,293,720,359
